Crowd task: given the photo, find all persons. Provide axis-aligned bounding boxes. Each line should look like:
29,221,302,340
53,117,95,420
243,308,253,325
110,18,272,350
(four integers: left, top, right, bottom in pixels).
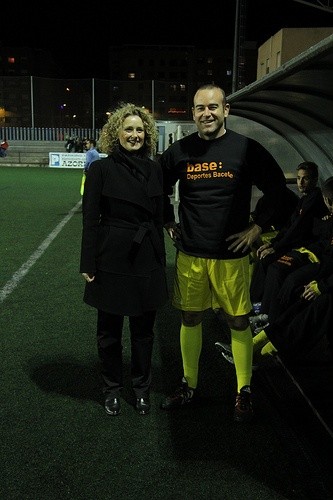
0,132,100,196
159,85,288,422
214,162,333,375
79,103,181,415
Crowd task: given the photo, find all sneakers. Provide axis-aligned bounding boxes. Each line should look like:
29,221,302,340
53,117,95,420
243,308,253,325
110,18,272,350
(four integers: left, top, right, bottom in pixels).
234,385,253,422
160,377,196,410
215,341,234,365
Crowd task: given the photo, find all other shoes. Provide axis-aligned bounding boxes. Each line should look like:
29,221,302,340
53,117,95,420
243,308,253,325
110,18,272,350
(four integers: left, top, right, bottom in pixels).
248,301,270,334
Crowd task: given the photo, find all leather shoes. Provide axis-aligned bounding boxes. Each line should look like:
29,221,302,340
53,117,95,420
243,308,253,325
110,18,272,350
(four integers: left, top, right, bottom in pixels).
135,396,151,415
103,391,120,417
